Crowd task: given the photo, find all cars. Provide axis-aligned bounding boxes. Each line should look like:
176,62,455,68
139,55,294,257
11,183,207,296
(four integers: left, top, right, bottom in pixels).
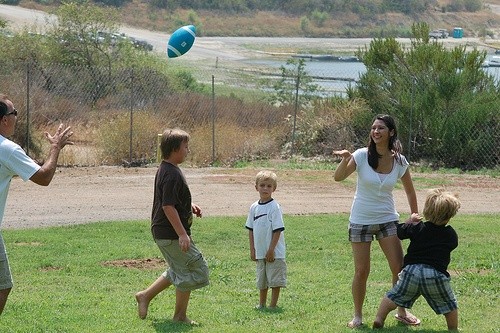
428,29,450,39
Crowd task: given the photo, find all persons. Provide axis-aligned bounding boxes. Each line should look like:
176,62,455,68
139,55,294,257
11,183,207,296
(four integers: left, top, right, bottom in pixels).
0,95,75,315
372,188,463,333
135,127,210,325
245,169,287,309
333,114,420,328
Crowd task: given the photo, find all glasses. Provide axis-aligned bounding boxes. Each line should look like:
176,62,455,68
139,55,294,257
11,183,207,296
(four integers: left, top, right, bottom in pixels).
1,108,17,118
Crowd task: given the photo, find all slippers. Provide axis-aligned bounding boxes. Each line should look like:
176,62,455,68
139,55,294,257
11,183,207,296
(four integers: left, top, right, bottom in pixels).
346,320,362,329
394,311,422,325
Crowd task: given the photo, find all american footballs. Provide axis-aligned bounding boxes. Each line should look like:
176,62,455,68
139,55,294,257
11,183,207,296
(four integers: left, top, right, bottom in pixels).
166,24,197,59
